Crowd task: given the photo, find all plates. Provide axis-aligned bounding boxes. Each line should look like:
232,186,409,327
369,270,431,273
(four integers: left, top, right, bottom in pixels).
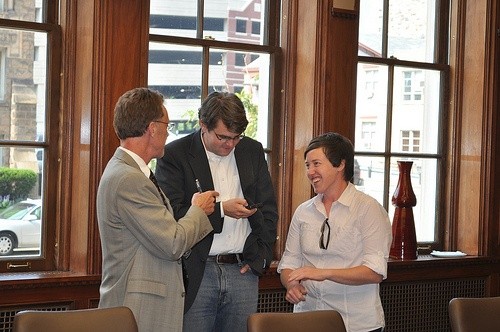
430,252,467,257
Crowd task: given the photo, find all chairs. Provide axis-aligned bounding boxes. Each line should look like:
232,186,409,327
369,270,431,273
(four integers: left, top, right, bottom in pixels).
246,309,347,332
448,296,500,332
11,306,138,332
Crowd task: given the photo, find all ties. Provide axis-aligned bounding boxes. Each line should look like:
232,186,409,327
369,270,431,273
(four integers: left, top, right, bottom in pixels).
149,170,189,295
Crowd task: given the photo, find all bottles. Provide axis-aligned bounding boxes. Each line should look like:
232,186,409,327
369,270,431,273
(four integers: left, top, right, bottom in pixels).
389,161,417,261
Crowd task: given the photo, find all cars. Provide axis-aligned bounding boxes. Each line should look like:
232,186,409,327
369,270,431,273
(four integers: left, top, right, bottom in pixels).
0,198,41,256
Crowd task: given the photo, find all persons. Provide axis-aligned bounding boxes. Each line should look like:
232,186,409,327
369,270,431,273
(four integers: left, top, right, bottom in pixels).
277,132,393,332
97,87,220,332
155,91,278,332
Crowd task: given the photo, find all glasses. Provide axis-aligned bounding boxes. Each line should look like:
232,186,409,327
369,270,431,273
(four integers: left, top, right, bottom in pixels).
320,218,331,250
209,123,245,142
145,120,174,131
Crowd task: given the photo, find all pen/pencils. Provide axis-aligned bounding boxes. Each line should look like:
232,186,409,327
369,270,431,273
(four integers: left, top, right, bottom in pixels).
195,178,203,193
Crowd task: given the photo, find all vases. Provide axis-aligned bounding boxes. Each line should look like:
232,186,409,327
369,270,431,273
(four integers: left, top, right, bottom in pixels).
389,160,419,261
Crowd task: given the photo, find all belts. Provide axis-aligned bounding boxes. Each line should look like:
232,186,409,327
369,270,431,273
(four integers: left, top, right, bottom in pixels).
207,253,244,264
